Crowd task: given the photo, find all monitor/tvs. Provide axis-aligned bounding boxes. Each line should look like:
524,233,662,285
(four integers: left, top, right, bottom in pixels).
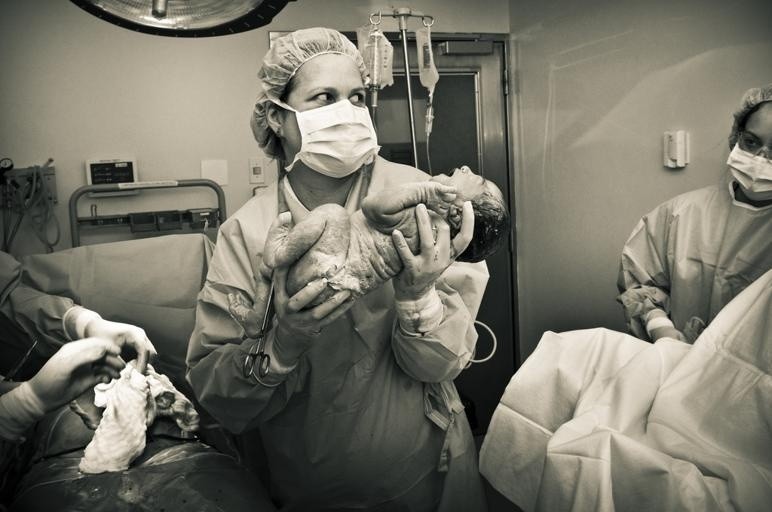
86,159,140,198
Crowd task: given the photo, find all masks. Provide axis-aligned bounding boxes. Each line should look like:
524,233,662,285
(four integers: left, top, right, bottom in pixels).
727,142,772,201
274,98,382,179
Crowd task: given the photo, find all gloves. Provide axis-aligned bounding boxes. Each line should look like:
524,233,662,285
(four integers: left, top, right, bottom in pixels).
248,263,355,389
390,200,476,337
0,301,159,442
642,306,688,345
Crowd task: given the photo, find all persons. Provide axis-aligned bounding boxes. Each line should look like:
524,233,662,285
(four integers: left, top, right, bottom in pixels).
185,27,490,512
0,249,158,471
227,166,509,338
616,85,772,343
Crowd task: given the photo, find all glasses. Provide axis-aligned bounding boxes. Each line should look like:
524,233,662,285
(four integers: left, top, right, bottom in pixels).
736,129,771,159
